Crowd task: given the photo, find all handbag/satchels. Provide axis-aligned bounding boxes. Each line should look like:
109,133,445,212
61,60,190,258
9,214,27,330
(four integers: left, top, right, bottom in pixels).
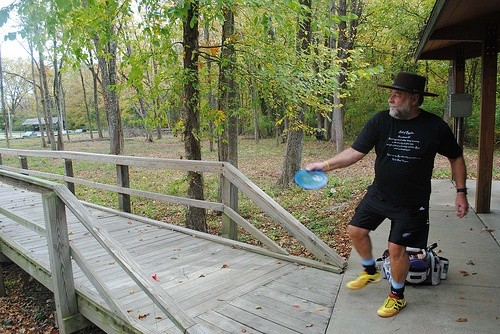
376,243,449,285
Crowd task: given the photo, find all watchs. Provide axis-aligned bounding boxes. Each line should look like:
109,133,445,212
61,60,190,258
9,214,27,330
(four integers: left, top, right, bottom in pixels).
456,188,468,194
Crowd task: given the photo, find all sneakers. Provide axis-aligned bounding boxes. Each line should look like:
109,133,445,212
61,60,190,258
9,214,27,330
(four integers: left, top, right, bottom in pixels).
346,270,382,289
377,293,407,317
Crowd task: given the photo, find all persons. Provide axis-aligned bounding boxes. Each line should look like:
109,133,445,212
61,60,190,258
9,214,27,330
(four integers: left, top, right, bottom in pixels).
305,74,469,318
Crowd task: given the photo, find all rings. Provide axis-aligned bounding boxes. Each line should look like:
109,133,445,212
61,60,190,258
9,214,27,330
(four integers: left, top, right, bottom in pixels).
465,210,469,213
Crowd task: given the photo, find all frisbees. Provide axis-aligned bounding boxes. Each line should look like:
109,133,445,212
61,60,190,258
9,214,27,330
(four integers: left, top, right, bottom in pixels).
295,169,329,190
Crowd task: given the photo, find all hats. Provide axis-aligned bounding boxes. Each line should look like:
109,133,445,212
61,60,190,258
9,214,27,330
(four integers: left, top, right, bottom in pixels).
377,72,439,97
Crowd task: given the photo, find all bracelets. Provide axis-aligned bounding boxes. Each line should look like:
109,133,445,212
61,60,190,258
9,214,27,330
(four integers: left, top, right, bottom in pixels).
324,160,329,171
